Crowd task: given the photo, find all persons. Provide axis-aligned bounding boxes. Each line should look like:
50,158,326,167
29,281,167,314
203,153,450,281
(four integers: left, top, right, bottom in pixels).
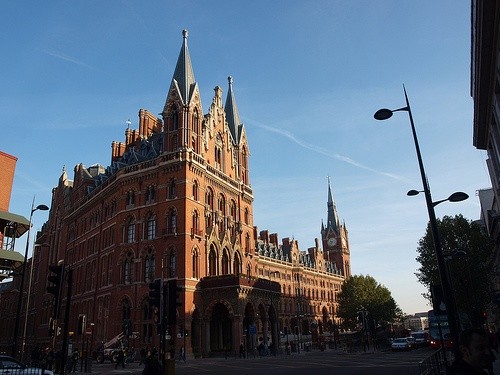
40,347,47,368
48,349,55,370
179,346,184,360
68,348,79,373
259,342,268,355
142,351,164,375
115,347,128,369
169,347,175,359
240,344,243,357
446,328,490,375
287,344,291,352
55,349,61,372
31,347,40,367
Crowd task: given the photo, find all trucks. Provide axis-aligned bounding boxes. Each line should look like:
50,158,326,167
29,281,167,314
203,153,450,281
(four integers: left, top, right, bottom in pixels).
412,331,428,345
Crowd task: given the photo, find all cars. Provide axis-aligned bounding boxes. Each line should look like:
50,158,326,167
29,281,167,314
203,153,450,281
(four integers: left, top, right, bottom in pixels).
0,355,53,375
392,336,414,352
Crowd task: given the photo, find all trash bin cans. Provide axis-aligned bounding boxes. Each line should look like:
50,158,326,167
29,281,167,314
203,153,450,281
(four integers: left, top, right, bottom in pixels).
165,359,175,375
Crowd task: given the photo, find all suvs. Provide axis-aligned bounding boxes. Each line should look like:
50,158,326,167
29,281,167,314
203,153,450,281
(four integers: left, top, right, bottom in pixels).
96,341,136,364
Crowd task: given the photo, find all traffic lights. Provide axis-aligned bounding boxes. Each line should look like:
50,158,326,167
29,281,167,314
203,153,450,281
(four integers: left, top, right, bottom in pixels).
47,264,63,295
164,279,186,309
148,279,161,309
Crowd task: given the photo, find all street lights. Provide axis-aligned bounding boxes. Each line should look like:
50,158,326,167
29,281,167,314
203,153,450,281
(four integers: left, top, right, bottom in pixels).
374,84,470,375
20,204,50,361
